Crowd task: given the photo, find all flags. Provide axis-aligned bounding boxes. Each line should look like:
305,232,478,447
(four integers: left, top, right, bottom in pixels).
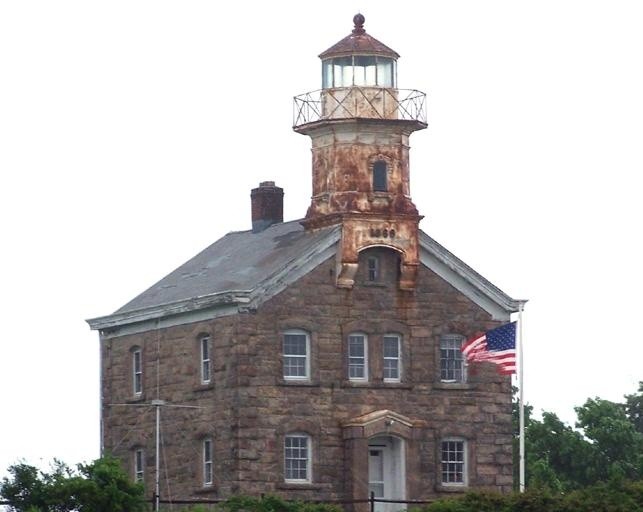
460,318,517,376
